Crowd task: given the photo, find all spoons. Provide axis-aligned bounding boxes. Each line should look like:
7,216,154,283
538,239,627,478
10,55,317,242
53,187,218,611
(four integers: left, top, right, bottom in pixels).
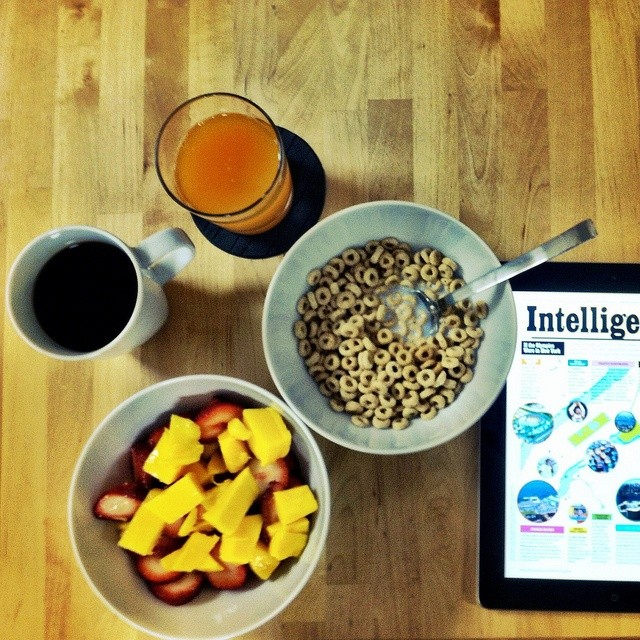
387,220,599,336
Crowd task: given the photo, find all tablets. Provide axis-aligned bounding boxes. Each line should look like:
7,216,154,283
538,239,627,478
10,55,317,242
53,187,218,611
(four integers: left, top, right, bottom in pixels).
479,261,640,613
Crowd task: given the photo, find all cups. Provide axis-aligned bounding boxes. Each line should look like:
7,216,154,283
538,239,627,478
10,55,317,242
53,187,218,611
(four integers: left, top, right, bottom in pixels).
156,93,295,235
7,226,196,362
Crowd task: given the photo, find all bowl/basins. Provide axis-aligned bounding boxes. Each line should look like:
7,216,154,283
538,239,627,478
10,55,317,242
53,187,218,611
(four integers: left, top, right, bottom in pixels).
261,200,518,456
68,372,332,636
